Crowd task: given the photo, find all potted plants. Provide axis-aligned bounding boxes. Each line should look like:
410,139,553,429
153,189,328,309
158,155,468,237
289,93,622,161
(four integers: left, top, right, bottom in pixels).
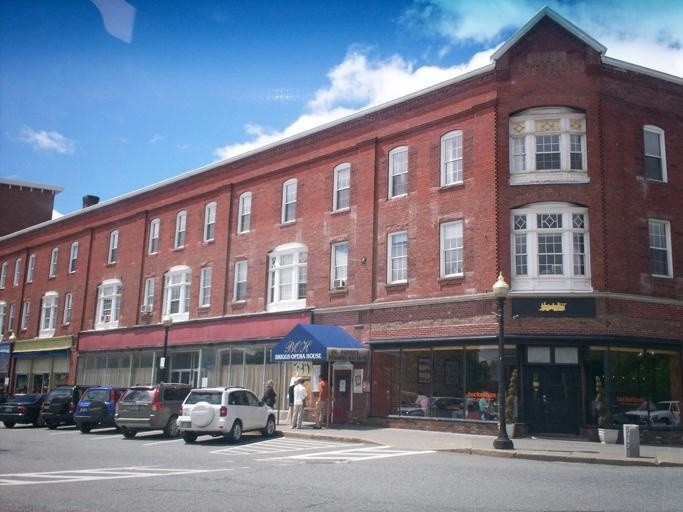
595,376,619,444
497,369,518,438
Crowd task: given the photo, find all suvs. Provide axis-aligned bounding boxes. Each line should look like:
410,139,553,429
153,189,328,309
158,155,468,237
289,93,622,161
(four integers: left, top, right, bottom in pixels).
73,386,127,432
115,383,195,438
393,389,493,416
40,383,100,430
628,399,680,425
174,386,278,443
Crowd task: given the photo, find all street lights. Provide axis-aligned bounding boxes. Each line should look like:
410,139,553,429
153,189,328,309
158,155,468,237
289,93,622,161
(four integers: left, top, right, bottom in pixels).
491,271,514,450
159,316,172,381
8,333,17,391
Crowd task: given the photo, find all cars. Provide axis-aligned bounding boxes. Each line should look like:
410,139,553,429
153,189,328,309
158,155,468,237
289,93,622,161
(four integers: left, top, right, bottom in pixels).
1,392,45,428
609,406,626,424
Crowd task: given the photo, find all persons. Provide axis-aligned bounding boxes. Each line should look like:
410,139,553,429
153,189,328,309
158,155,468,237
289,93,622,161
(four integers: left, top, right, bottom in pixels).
285,376,297,424
260,380,277,411
311,373,327,428
430,388,498,418
288,377,307,428
410,391,431,417
